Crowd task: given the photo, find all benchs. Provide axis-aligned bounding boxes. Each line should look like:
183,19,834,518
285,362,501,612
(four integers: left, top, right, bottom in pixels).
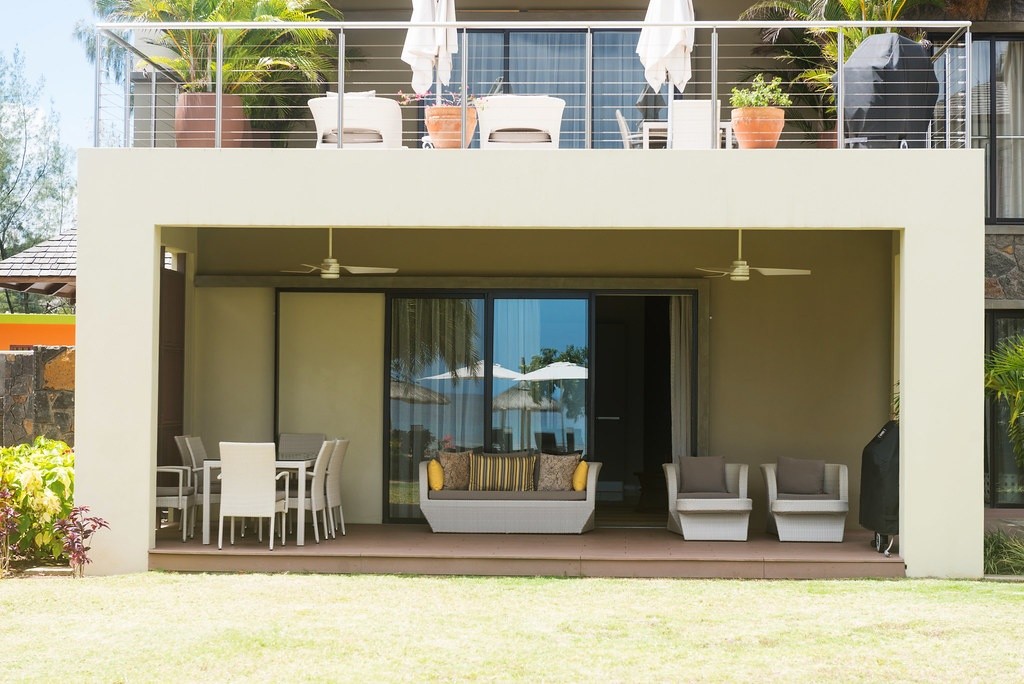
201,449,320,546
641,120,732,149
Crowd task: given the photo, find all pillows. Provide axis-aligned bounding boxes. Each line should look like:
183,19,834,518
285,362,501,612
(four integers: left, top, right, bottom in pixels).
678,452,730,493
481,451,530,456
572,460,588,490
468,452,538,491
428,457,444,490
777,454,826,495
325,89,376,97
538,452,580,491
435,449,474,489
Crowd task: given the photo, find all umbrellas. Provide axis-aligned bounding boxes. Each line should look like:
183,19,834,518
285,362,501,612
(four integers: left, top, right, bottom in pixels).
401,0,458,106
416,360,523,379
493,380,562,449
391,377,450,405
513,362,588,450
636,0,694,149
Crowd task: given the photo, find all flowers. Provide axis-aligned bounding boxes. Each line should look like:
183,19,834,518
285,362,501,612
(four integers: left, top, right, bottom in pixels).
398,86,488,110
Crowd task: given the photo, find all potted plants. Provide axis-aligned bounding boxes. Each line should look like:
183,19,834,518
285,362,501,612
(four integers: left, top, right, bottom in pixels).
108,0,374,152
730,70,794,149
735,0,924,148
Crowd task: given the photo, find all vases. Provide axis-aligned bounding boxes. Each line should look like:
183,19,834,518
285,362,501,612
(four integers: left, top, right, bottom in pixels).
424,104,477,149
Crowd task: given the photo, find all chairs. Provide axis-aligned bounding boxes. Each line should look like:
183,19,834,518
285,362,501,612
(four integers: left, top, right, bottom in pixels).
279,440,335,544
279,432,328,526
307,95,403,148
672,99,724,149
174,434,196,535
156,465,196,544
185,436,246,545
476,94,567,149
615,109,667,149
289,438,352,540
216,441,290,551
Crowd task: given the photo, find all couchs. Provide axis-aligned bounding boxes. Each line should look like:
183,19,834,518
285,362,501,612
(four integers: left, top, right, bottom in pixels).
759,462,850,543
661,461,756,542
418,460,602,535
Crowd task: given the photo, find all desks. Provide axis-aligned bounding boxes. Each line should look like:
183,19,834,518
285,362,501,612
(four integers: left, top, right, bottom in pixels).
421,135,434,149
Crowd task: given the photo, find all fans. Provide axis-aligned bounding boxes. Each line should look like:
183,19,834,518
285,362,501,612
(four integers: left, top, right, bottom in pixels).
279,228,400,281
695,229,811,284
